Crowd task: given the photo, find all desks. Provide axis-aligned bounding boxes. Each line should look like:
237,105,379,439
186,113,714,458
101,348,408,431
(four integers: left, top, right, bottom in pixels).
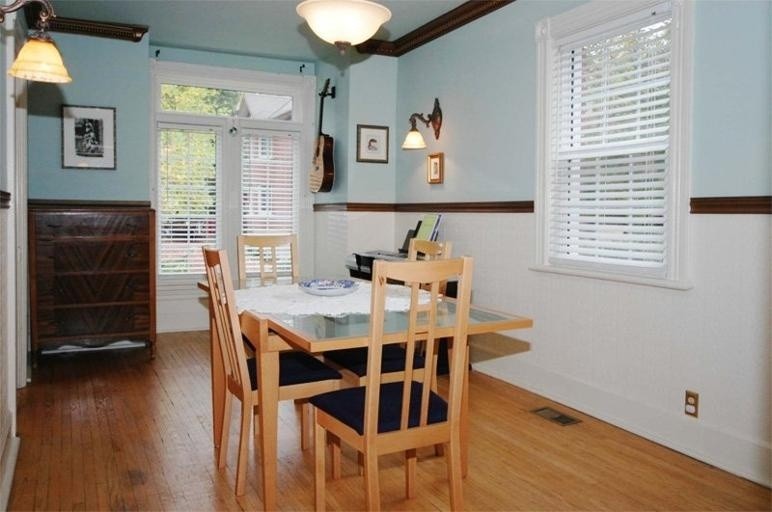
345,250,459,352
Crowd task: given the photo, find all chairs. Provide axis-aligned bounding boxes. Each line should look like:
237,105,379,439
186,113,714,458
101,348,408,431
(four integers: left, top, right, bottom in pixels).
236,234,298,415
351,253,376,281
314,255,475,512
202,243,343,496
324,240,451,476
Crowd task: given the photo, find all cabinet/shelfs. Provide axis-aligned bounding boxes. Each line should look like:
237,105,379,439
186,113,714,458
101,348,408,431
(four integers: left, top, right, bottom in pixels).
27,201,157,363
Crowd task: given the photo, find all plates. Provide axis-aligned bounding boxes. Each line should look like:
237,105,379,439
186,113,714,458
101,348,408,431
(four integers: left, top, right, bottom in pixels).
297,278,360,297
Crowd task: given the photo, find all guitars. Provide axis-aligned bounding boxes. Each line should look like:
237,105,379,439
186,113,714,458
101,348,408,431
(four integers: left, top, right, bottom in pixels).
309,78,334,193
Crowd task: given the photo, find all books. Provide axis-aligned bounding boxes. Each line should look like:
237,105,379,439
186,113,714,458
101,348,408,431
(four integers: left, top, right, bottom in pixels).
397,213,443,257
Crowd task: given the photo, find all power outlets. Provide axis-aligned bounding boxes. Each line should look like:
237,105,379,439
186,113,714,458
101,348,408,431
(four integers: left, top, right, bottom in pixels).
684,389,699,418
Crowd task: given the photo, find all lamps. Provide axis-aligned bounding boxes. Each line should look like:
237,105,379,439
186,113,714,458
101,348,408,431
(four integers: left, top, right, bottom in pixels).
0,0,72,84
402,98,442,149
296,0,392,56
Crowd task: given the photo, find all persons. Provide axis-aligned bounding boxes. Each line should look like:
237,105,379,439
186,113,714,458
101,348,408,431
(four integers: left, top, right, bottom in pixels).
368,138,378,151
82,123,98,153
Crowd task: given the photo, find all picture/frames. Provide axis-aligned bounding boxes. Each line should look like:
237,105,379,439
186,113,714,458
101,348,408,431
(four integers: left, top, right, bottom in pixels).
357,124,389,163
428,152,444,184
60,104,116,170
